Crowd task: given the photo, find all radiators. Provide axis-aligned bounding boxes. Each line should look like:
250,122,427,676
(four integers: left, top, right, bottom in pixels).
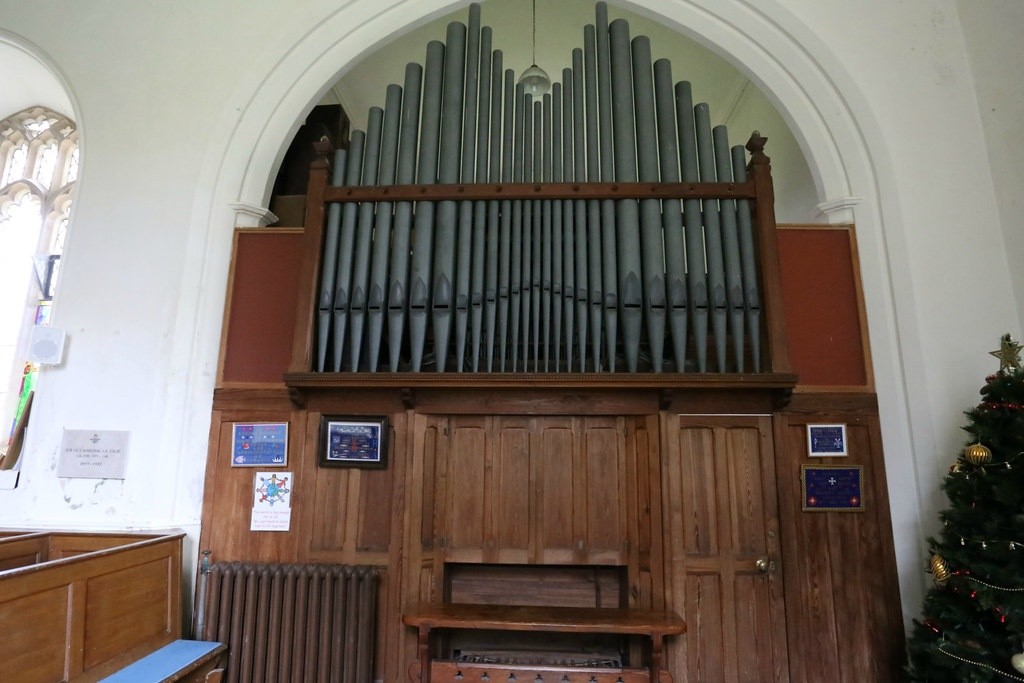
203,562,387,683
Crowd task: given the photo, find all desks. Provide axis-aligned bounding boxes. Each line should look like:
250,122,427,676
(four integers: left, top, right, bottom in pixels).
403,602,686,683
97,639,225,683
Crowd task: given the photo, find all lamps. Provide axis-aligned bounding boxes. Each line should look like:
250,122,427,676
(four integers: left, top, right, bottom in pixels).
518,1,551,95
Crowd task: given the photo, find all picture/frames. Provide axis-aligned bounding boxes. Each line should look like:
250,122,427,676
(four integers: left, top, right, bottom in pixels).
801,463,865,512
319,414,390,468
231,421,288,467
805,422,849,458
